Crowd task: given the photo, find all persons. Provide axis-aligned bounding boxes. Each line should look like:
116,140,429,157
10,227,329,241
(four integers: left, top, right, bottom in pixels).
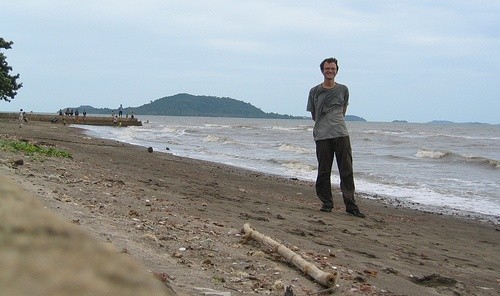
306,58,366,219
16,101,142,128
